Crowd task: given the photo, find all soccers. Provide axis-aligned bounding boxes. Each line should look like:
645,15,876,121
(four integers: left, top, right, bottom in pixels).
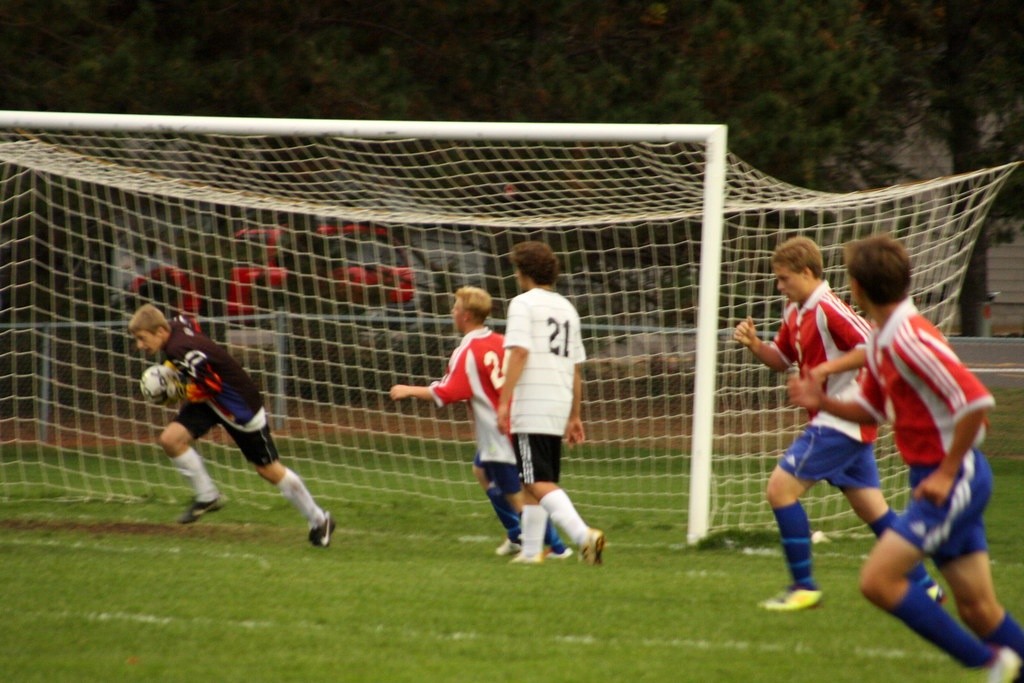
138,364,182,407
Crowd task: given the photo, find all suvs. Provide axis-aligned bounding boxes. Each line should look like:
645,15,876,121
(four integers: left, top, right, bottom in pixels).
119,221,414,342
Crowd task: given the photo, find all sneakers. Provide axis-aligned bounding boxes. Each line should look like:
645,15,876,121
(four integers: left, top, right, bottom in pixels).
756,586,824,612
495,534,523,556
307,510,339,547
926,584,948,606
508,552,545,565
543,545,575,560
177,494,225,524
985,647,1023,683
578,528,607,568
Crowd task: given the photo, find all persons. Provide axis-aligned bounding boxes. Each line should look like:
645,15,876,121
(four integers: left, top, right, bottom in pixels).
128,303,336,548
734,235,946,613
389,286,573,561
789,236,1024,683
497,240,605,565
